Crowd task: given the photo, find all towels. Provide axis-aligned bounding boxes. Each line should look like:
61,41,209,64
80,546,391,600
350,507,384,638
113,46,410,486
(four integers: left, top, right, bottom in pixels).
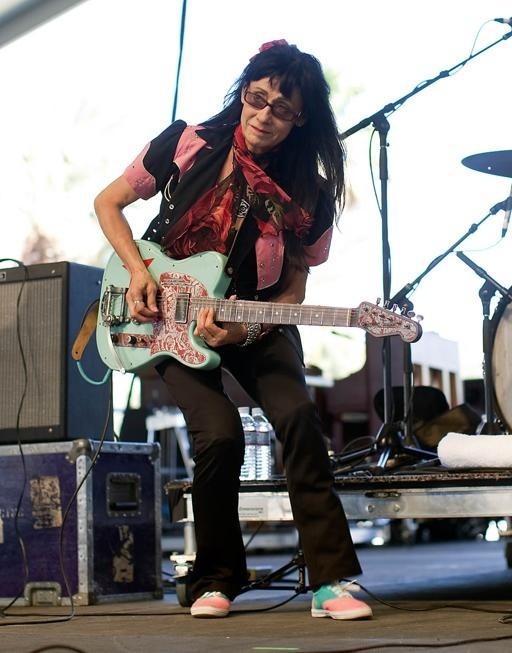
436,431,512,471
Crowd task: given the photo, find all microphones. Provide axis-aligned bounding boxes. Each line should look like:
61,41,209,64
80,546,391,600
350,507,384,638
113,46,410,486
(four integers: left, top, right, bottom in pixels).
494,14,512,29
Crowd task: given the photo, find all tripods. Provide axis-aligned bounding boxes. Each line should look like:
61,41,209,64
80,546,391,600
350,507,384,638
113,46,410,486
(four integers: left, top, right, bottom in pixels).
329,33,512,474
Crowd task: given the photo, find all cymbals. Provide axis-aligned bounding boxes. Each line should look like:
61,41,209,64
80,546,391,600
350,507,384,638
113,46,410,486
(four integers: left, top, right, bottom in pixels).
461,150,510,177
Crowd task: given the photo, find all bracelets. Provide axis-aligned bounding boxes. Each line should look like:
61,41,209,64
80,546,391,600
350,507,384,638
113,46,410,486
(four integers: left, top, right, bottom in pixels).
243,322,261,349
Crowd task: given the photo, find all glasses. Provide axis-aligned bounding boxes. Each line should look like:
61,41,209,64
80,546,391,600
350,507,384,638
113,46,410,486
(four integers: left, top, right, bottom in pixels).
243,85,302,124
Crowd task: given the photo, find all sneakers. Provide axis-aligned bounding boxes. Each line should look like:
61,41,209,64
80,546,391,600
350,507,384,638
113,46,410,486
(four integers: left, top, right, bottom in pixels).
190,590,231,619
309,584,372,622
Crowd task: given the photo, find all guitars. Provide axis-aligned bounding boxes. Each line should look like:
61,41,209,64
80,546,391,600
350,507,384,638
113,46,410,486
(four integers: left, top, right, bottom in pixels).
95,241,422,372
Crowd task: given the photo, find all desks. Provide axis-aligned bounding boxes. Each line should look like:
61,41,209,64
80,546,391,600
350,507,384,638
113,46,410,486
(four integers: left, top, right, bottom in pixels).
165,470,512,608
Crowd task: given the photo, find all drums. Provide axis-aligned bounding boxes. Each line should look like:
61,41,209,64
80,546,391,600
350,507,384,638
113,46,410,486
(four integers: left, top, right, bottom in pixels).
489,285,512,434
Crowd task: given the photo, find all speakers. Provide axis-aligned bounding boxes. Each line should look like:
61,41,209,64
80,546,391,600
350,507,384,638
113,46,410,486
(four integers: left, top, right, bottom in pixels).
2,259,113,442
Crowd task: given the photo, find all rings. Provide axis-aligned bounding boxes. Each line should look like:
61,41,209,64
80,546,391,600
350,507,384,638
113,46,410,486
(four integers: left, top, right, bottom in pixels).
134,299,143,304
199,335,206,341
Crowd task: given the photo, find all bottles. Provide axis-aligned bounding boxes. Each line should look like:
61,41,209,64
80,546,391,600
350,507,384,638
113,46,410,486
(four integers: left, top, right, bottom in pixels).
238,406,257,481
251,406,272,480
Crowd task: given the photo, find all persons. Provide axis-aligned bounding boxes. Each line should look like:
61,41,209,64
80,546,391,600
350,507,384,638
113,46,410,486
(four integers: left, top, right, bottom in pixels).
95,39,374,621
